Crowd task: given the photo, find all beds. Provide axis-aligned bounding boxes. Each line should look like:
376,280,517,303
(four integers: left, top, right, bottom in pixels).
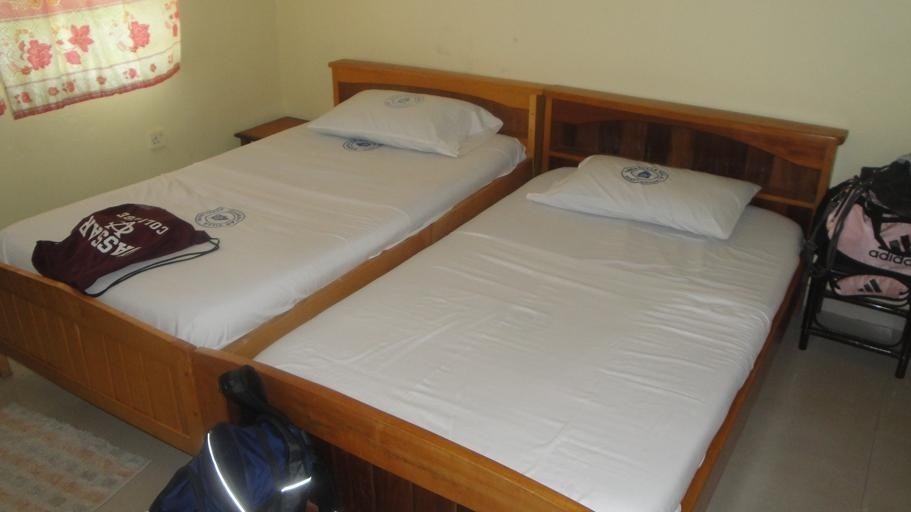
194,83,850,512
1,58,548,454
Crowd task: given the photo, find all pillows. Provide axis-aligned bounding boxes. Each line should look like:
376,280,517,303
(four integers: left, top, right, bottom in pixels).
306,88,504,158
526,154,763,241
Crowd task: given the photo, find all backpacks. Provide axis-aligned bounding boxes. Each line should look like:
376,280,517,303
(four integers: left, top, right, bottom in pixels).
809,158,911,307
150,364,338,511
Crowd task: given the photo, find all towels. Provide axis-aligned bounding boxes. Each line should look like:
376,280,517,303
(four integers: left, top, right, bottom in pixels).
0,403,153,512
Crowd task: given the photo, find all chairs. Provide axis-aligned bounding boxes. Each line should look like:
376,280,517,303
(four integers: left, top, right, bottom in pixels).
799,166,911,379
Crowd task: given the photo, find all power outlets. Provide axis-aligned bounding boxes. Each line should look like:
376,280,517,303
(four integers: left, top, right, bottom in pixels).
147,133,165,149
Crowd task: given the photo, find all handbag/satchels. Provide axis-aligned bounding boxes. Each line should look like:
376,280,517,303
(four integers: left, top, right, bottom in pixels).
31,204,222,298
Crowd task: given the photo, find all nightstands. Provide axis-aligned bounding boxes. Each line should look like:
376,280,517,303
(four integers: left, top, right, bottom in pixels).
234,116,309,145
